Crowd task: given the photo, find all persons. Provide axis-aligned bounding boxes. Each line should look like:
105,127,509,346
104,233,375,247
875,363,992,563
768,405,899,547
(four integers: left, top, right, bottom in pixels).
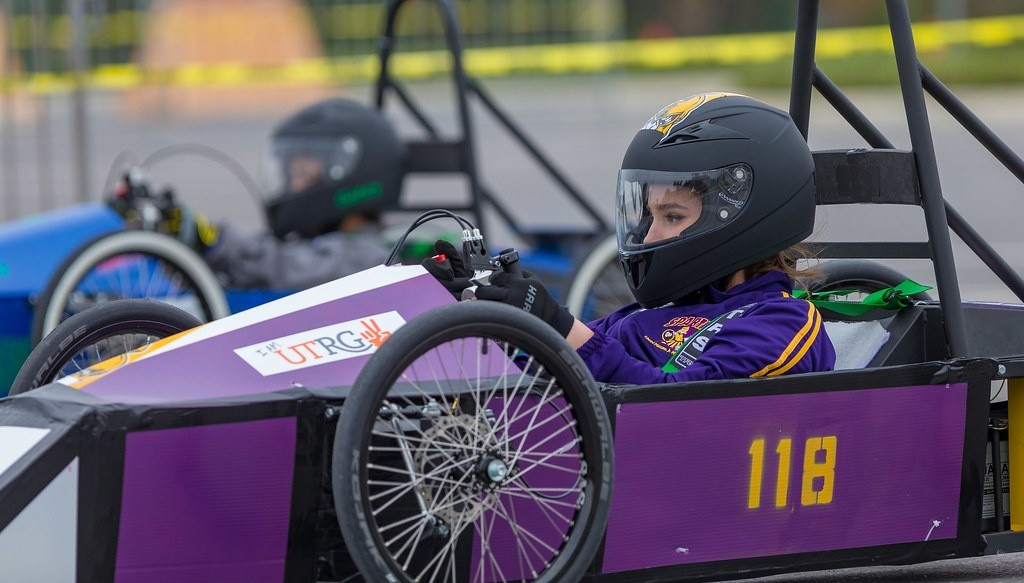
106,97,407,288
421,91,837,385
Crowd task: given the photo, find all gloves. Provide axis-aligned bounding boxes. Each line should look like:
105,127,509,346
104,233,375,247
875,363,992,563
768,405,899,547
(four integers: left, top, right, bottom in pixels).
420,241,474,302
475,270,575,339
109,170,219,266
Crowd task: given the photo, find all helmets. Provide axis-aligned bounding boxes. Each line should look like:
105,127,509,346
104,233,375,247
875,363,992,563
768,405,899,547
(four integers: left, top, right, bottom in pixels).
264,97,404,242
614,92,815,309
